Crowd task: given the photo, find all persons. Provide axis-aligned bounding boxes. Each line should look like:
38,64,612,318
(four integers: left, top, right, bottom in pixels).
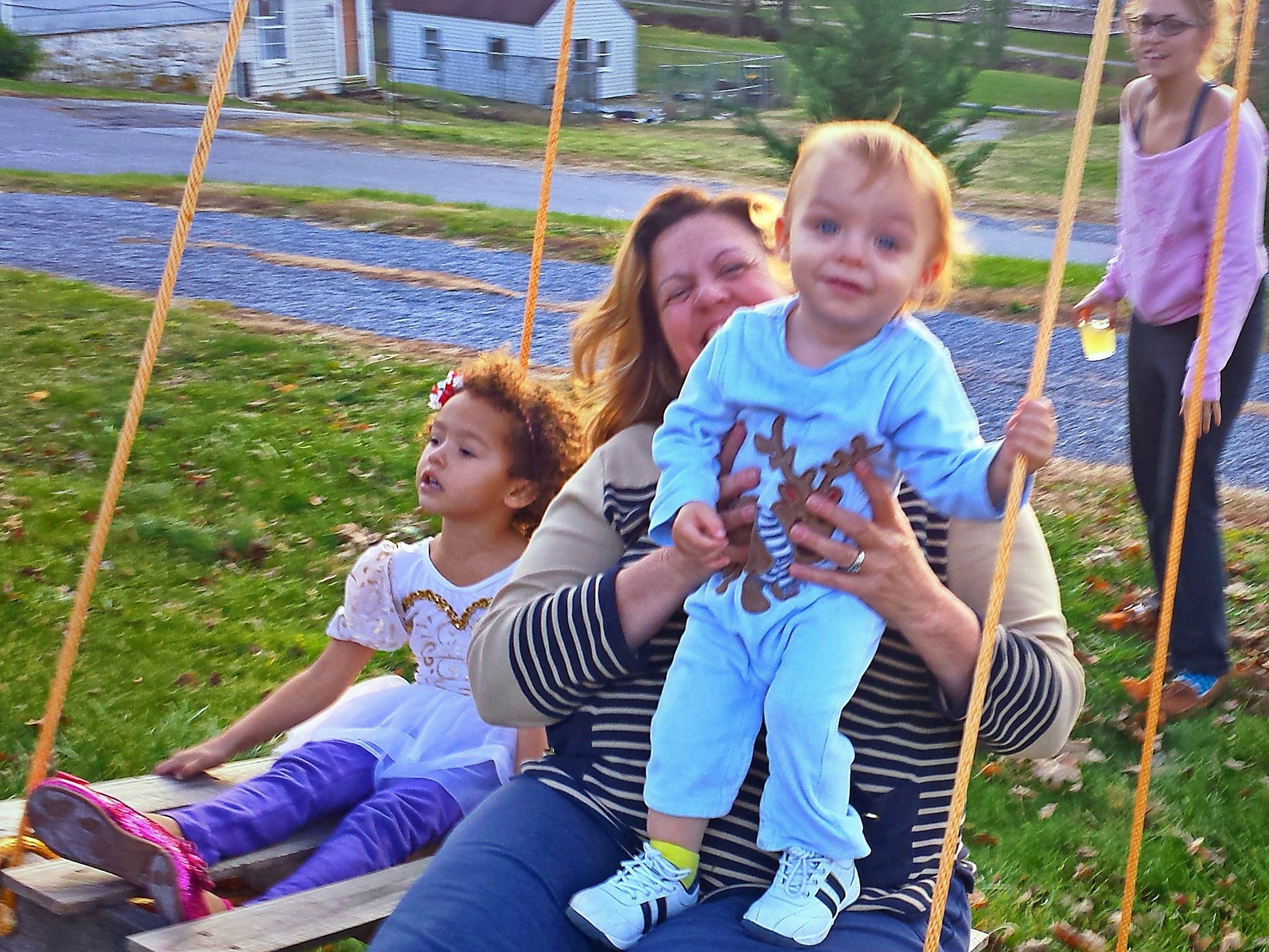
1071,0,1269,716
365,185,1086,952
569,121,1056,952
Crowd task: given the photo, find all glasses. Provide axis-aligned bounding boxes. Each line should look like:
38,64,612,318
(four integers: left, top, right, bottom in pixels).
1126,15,1209,37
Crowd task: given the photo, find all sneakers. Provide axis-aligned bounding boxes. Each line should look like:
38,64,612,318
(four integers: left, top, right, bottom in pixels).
567,842,700,952
742,844,862,944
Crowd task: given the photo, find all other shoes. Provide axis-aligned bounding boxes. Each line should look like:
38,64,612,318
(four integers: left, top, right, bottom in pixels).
25,778,200,887
145,849,235,923
1159,670,1226,721
1095,605,1163,638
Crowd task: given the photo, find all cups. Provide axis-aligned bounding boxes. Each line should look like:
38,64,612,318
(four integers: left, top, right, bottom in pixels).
1077,314,1116,361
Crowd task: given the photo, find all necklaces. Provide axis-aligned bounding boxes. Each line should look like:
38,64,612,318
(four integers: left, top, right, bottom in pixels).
25,349,584,928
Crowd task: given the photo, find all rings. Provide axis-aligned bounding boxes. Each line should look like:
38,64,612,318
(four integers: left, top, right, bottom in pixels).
844,551,867,575
